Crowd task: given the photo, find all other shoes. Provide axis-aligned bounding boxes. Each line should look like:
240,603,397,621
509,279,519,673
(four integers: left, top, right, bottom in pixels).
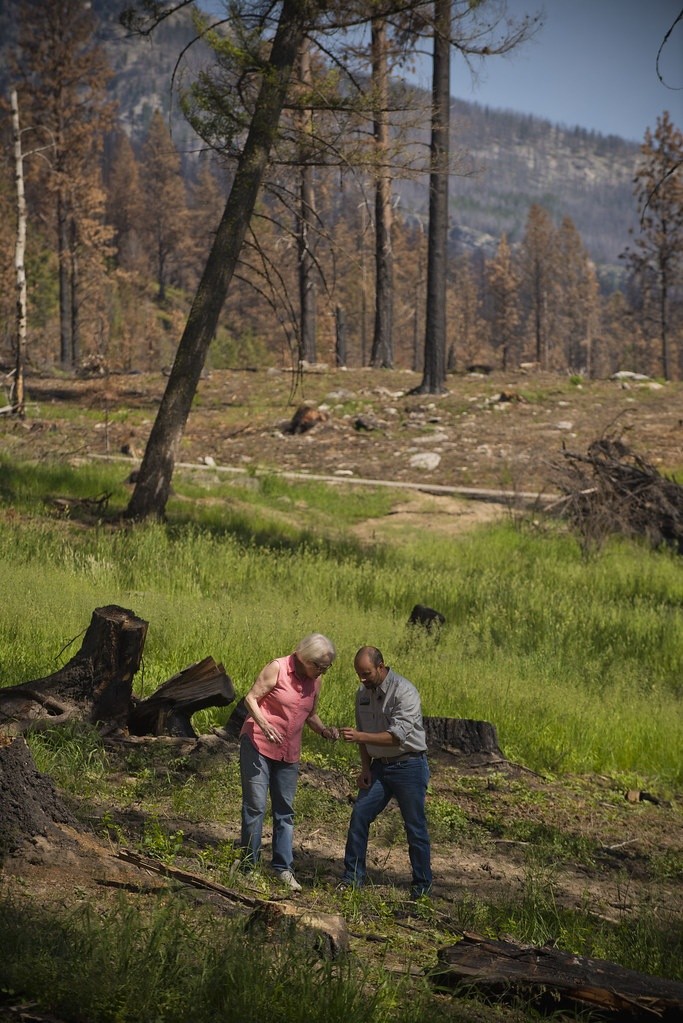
410,882,432,903
246,871,266,892
276,870,303,892
339,879,366,888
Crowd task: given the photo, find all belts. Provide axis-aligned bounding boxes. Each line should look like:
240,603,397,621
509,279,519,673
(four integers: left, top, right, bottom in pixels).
377,751,426,765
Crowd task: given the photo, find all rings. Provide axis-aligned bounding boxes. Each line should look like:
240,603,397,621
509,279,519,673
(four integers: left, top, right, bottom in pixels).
270,736,274,740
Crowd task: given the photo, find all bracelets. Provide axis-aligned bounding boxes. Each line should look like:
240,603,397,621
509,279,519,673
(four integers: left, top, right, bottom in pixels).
321,729,328,739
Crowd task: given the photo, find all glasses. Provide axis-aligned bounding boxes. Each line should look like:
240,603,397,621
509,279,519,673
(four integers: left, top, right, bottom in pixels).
313,662,333,670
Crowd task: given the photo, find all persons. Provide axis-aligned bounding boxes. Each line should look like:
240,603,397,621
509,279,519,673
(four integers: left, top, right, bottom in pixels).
335,645,432,904
238,632,340,893
